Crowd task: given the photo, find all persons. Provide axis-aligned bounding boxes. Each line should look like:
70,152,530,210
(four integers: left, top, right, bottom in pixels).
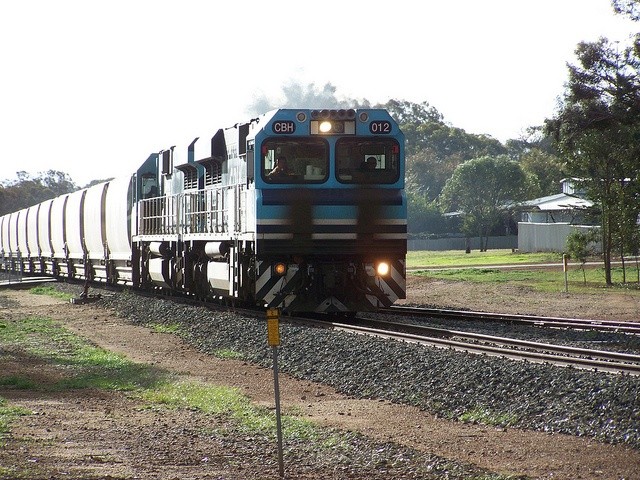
362,157,380,178
267,156,290,177
149,186,157,195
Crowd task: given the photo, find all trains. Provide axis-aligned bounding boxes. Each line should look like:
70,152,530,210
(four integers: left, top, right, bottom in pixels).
0,109,408,311
